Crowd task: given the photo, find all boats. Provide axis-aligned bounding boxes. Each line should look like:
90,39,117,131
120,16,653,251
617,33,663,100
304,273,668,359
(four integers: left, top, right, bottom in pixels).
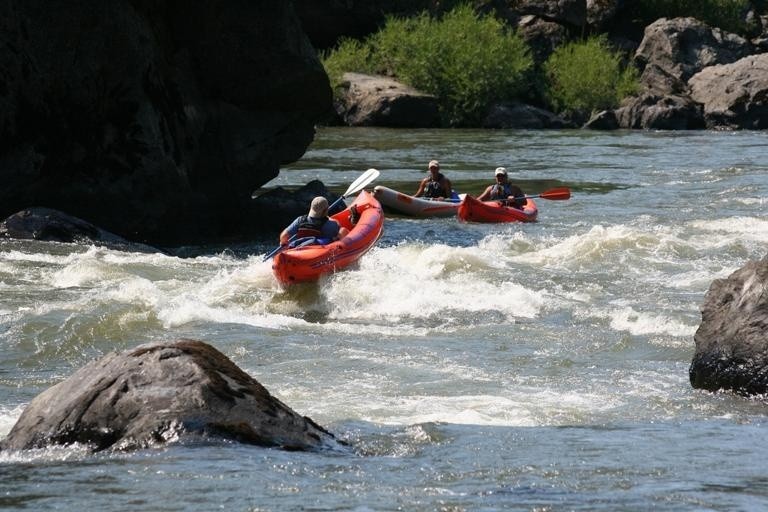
372,186,537,223
272,185,384,287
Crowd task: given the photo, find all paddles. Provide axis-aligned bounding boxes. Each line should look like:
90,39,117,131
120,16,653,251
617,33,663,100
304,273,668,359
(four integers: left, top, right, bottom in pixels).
262,168,380,262
500,187,570,201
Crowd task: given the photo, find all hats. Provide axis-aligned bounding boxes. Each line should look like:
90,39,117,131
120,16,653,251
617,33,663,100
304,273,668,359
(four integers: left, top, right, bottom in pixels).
495,167,507,176
429,160,440,168
309,196,328,218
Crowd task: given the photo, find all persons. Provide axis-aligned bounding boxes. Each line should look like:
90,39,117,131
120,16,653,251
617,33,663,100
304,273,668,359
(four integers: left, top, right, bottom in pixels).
412,160,451,201
477,166,527,210
279,197,349,250
